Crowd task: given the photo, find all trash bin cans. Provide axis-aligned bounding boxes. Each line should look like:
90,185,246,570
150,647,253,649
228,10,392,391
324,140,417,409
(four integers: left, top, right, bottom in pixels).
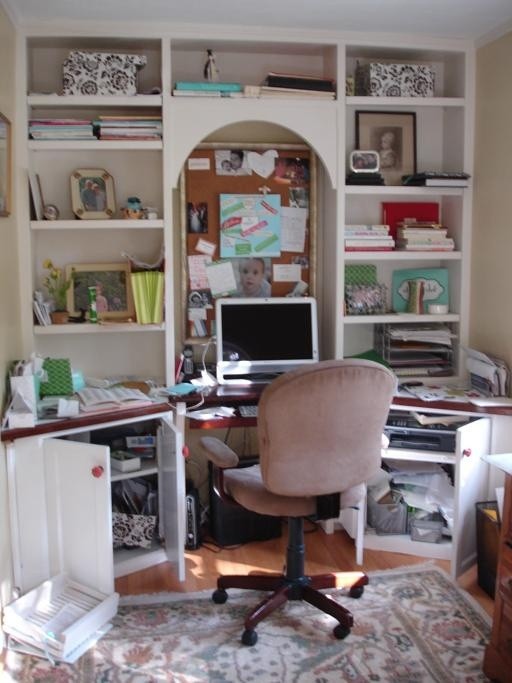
475,500,501,601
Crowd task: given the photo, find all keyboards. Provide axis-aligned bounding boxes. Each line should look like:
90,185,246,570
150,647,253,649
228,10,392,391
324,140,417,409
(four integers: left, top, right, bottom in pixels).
239,405,257,418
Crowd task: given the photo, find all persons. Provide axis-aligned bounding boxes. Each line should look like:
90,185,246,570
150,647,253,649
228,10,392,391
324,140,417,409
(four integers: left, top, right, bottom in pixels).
230,257,271,297
220,150,249,176
354,129,401,169
96,284,108,312
81,179,106,211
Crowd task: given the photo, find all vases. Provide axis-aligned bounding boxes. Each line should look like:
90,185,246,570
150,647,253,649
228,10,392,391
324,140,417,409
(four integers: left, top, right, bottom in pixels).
49,308,69,324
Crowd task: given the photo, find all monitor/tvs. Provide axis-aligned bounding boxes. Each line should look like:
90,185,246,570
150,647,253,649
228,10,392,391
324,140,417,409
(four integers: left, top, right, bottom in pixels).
215,297,319,385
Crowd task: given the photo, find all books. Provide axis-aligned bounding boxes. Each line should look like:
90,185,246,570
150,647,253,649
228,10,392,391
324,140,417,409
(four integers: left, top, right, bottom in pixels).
411,410,471,425
400,172,468,187
28,173,46,219
76,387,151,413
344,221,455,251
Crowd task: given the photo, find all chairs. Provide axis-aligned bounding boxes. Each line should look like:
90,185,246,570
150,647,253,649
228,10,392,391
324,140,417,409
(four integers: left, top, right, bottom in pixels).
200,354,399,648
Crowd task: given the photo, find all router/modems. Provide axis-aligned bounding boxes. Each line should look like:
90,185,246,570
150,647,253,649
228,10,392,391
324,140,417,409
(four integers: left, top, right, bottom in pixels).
186,488,200,549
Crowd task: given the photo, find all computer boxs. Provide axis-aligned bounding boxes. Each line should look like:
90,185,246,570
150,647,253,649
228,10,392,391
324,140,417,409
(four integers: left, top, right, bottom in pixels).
208,454,282,546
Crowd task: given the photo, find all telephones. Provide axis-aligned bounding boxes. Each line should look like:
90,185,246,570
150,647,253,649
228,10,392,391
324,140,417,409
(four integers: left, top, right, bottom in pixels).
183,345,197,382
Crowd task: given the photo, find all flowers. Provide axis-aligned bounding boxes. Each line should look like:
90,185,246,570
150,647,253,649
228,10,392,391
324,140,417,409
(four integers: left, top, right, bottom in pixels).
42,258,72,310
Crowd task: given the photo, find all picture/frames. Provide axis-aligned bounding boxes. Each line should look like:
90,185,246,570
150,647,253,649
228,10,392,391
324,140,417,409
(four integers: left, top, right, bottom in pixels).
0,111,13,221
68,165,116,218
65,259,132,318
355,110,420,185
179,136,320,350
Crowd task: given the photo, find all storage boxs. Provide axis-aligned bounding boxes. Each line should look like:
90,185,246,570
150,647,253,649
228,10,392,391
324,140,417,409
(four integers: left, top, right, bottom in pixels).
61,49,147,97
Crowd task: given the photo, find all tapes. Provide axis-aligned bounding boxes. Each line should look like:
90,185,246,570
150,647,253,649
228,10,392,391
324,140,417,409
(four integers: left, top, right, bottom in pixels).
428,303,450,314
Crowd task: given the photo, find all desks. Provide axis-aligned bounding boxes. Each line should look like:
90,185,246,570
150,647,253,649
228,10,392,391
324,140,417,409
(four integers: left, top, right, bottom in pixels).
174,387,338,581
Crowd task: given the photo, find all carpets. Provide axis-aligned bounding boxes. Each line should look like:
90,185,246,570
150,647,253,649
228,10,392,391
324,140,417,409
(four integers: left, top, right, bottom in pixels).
1,561,511,681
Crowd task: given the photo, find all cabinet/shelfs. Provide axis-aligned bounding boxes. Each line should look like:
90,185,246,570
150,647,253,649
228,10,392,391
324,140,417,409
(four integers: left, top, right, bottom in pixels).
0,407,188,591
336,30,479,386
341,388,512,582
12,22,168,402
479,451,512,683
353,62,436,96
166,30,340,104
